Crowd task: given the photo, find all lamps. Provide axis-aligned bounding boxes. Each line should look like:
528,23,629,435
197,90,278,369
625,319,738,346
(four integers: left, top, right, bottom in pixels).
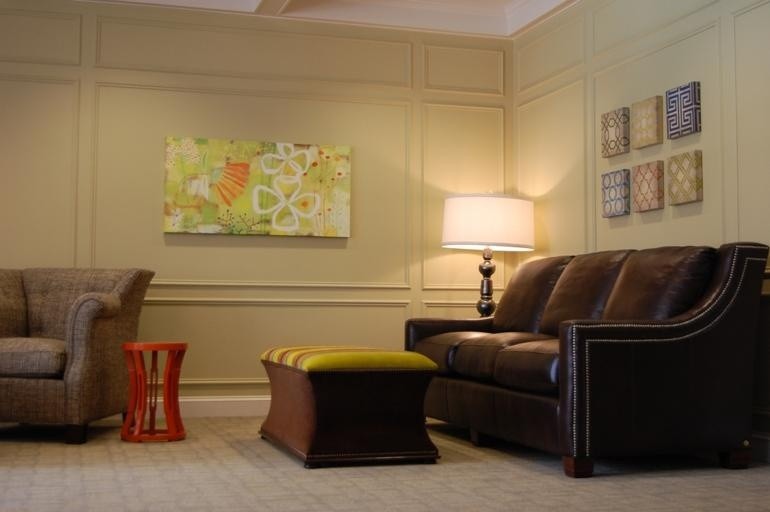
440,194,537,322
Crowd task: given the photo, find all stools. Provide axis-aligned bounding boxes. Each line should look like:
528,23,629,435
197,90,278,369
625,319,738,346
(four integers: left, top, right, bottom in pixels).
120,341,187,445
257,347,440,468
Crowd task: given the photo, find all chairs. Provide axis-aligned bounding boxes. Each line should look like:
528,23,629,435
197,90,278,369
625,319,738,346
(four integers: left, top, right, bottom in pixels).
0,266,156,443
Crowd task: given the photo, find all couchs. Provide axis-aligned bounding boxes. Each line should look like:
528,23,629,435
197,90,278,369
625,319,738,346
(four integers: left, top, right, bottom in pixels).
404,241,770,477
